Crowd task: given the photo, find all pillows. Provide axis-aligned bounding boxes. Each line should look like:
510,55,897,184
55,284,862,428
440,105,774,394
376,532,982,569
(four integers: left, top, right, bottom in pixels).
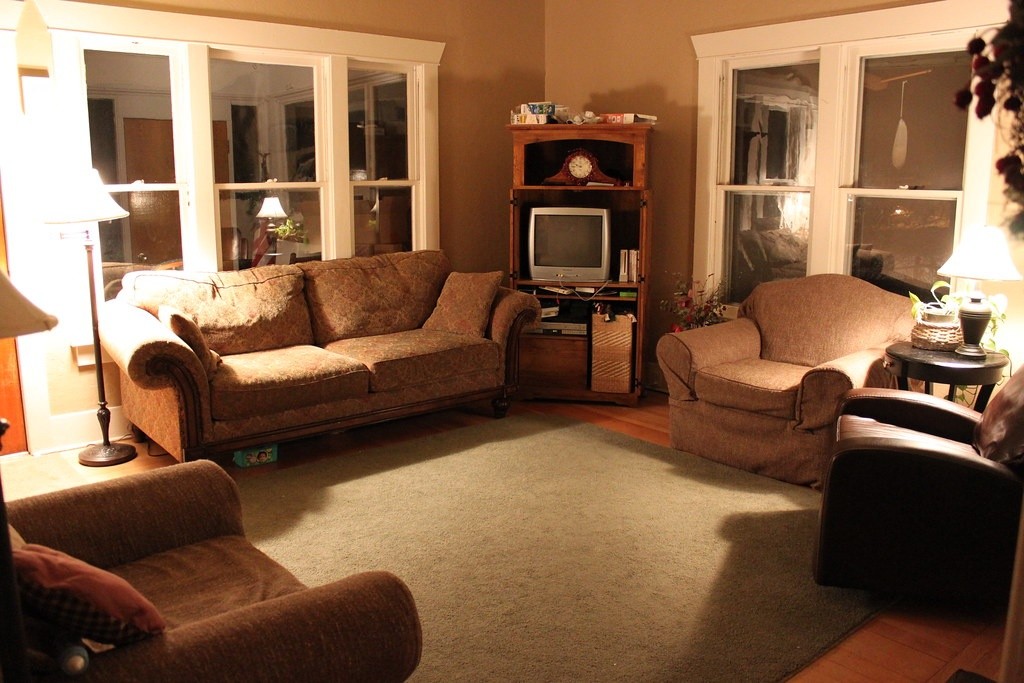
159,302,218,379
4,524,171,647
422,268,503,341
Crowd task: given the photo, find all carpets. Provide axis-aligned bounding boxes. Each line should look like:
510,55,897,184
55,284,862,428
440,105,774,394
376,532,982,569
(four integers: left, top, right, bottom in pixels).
1,406,905,679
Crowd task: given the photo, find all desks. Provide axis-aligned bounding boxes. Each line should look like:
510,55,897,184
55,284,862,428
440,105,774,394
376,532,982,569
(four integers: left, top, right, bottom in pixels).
886,337,1010,412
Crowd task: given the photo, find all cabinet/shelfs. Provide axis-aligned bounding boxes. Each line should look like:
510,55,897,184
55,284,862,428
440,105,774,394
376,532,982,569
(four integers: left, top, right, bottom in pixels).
500,104,663,408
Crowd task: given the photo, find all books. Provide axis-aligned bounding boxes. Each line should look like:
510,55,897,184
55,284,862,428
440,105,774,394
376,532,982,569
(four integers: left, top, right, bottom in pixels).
618,248,640,284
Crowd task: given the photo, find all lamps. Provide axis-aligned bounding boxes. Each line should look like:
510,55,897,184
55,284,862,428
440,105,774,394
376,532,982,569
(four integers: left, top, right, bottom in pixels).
55,163,140,470
256,197,290,262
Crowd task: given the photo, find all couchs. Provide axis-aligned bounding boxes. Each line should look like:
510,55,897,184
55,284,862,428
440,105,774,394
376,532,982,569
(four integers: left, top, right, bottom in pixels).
812,360,1024,623
2,459,426,683
656,271,936,485
95,246,542,468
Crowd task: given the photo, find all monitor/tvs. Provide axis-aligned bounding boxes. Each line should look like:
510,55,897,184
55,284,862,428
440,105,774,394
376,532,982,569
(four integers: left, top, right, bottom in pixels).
529,207,611,282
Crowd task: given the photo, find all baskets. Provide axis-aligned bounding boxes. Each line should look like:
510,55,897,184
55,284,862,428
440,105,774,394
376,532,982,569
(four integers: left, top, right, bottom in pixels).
911,320,964,352
